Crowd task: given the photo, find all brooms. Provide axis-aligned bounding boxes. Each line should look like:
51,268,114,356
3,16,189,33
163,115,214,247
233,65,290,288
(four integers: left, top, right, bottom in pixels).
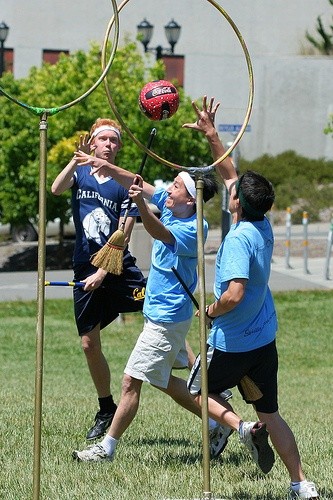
169,265,263,402
89,128,158,276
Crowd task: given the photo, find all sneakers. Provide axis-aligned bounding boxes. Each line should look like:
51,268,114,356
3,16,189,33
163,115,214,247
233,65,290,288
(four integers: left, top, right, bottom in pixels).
72,443,114,463
208,422,234,460
290,482,319,500
87,403,117,441
240,421,274,474
220,389,233,400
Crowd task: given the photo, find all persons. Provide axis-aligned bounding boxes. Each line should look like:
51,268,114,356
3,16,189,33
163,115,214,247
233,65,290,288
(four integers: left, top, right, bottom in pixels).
68,148,276,474
54,119,233,443
181,96,320,499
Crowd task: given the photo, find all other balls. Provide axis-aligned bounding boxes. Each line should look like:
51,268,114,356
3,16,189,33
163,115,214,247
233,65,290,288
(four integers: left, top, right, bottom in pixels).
139,81,180,122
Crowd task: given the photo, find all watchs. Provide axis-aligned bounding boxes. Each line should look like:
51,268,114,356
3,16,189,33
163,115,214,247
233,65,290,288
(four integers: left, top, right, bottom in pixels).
204,305,216,319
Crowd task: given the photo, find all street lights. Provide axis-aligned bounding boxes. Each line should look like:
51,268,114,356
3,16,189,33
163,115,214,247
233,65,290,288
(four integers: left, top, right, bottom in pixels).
138,16,182,80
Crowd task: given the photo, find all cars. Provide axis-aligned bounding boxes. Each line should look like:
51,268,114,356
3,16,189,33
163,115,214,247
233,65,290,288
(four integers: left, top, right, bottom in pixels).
0,206,76,245
